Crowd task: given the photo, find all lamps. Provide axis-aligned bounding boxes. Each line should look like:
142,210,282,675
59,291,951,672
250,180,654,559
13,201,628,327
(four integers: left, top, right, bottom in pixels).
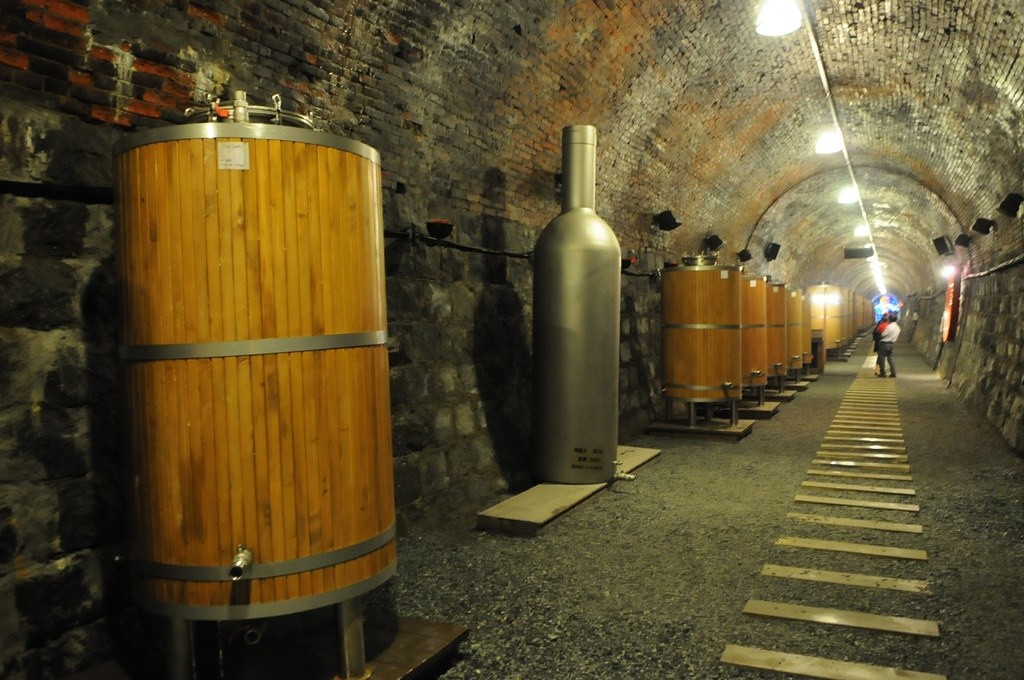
842,246,873,258
954,232,972,249
972,217,994,234
701,234,727,253
737,246,754,262
994,193,1023,218
932,236,952,256
764,243,782,261
648,207,684,233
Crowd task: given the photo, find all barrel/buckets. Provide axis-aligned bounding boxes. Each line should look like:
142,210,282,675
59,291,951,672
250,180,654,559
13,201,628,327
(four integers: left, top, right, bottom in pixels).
655,243,875,416
100,95,399,617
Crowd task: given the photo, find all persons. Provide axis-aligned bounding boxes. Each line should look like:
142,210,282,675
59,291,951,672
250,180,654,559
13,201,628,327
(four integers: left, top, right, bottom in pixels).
873,312,901,377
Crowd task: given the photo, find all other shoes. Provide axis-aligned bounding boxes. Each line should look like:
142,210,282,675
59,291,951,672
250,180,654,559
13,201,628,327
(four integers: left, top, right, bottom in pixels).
889,374,896,378
879,372,886,377
